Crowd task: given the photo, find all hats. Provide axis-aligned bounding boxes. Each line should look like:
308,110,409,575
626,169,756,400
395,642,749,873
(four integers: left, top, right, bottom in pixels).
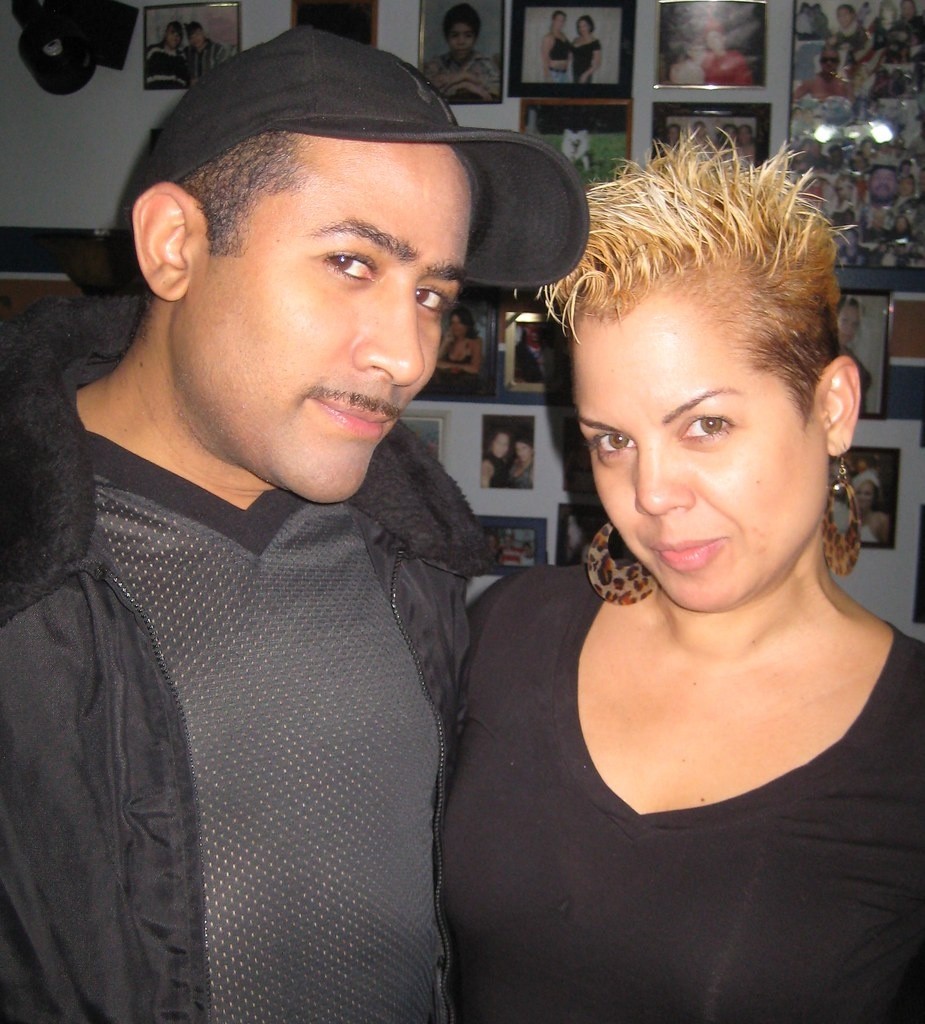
125,27,590,287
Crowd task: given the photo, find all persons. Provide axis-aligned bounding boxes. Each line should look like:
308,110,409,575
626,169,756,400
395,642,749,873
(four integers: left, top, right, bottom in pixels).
658,2,923,271
437,121,924,1024
569,14,602,83
846,469,890,546
179,20,230,89
827,457,855,535
831,291,874,416
0,23,588,1021
422,3,500,102
433,292,621,571
145,20,191,88
541,9,570,82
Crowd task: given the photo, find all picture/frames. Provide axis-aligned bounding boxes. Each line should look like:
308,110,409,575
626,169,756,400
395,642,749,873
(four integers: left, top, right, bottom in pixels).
1,0,925,625
